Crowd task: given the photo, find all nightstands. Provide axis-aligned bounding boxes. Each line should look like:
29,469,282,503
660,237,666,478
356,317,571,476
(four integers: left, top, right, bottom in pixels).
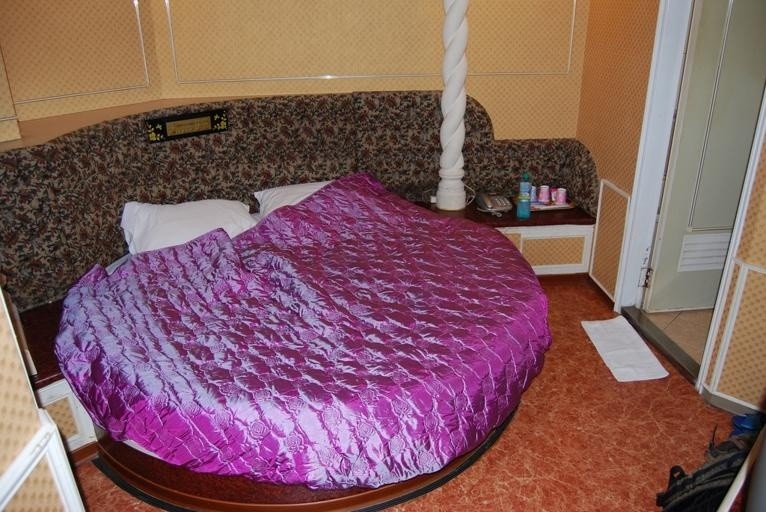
17,296,99,460
408,192,597,277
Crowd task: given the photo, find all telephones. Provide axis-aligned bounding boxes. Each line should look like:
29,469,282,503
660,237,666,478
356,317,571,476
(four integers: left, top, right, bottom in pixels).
475,192,512,213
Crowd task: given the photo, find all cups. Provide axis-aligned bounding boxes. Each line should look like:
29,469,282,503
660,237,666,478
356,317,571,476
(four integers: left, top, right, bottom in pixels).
532,185,551,202
555,188,568,205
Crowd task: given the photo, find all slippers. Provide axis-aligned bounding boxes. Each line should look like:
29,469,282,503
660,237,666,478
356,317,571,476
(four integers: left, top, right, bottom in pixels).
729,412,763,437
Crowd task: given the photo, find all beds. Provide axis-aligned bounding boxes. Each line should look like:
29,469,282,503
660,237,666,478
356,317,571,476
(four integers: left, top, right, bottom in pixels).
0,87,602,511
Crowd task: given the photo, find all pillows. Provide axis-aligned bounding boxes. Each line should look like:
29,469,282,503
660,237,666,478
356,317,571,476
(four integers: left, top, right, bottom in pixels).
119,177,338,257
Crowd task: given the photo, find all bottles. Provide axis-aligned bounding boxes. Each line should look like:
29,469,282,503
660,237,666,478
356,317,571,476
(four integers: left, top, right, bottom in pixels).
517,173,531,221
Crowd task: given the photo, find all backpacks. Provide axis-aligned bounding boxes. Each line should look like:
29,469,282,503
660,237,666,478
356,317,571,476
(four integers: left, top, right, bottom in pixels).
655,424,753,512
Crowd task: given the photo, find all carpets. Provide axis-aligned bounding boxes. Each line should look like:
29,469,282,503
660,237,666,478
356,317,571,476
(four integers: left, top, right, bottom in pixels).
580,313,671,384
